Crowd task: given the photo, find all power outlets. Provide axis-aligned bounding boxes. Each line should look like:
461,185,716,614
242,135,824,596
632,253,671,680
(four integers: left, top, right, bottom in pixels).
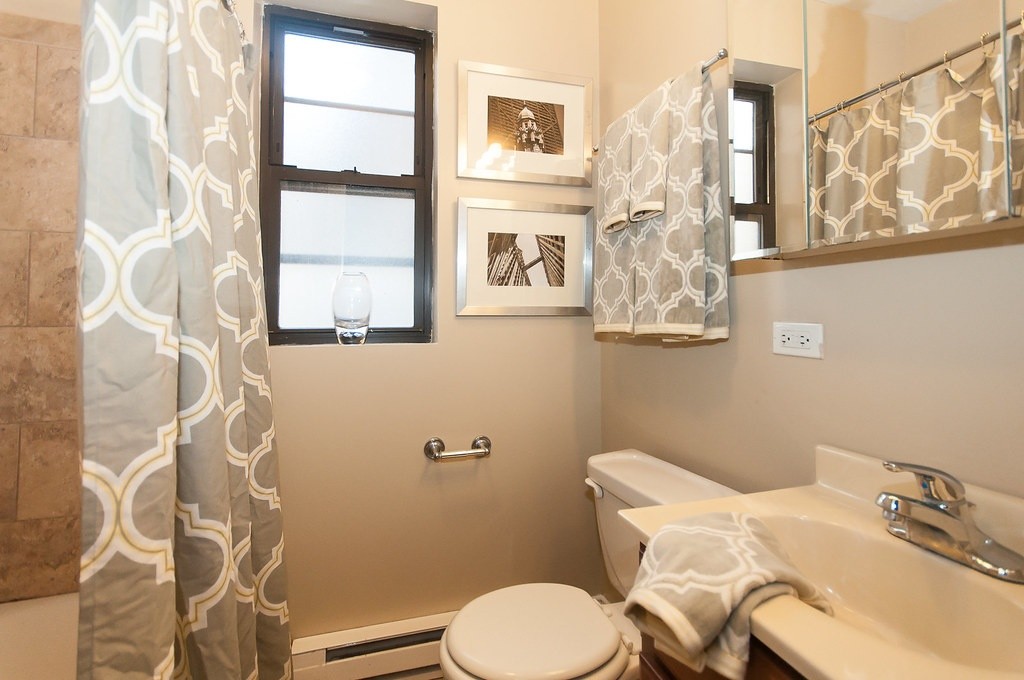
772,322,824,361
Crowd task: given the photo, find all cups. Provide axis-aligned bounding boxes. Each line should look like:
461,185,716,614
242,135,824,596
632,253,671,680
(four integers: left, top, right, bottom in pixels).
333,272,369,344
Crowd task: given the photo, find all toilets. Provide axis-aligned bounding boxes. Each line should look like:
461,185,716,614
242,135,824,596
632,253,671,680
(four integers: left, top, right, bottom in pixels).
438,442,756,680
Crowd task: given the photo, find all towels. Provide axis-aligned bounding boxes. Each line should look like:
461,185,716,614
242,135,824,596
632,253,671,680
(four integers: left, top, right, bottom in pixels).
619,514,836,674
584,64,742,345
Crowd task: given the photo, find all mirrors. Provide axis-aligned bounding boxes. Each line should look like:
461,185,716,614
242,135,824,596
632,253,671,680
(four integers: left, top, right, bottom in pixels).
720,0,1023,265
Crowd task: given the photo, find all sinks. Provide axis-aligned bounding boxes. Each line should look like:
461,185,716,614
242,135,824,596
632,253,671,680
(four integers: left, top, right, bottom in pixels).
619,443,1023,678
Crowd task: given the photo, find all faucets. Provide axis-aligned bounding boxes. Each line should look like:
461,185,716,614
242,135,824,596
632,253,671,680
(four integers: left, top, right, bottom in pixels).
877,460,1022,584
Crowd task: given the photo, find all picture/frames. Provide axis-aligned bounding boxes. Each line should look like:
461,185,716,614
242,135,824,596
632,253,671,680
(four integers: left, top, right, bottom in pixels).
452,196,596,317
455,59,595,187
